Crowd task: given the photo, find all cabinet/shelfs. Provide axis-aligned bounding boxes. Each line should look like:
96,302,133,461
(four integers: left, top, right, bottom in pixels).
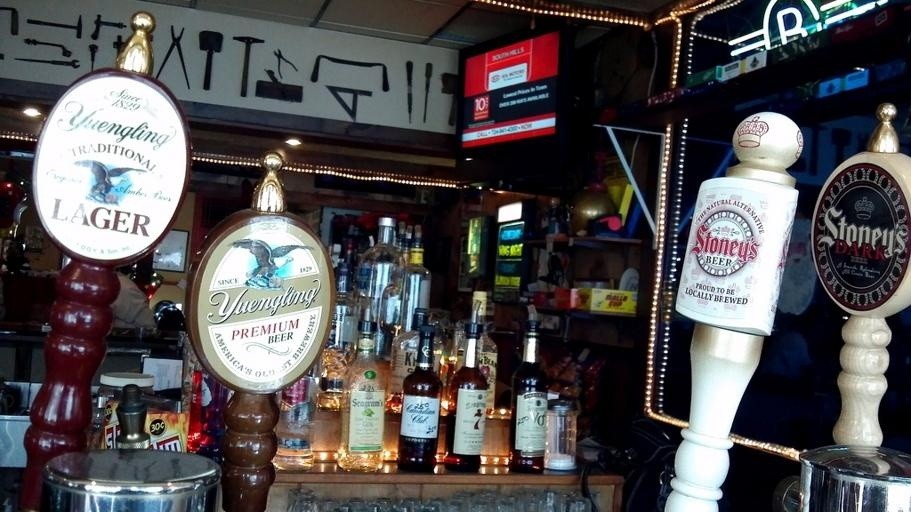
517,236,643,319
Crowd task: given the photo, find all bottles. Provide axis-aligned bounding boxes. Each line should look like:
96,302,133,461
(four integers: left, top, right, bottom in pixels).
87,369,155,451
272,213,583,477
12,175,63,273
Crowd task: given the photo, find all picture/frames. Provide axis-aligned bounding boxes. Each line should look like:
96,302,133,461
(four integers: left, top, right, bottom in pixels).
151,227,189,273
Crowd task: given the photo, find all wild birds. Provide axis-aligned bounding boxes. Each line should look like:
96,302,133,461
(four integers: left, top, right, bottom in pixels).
228,236,315,279
73,159,146,193
